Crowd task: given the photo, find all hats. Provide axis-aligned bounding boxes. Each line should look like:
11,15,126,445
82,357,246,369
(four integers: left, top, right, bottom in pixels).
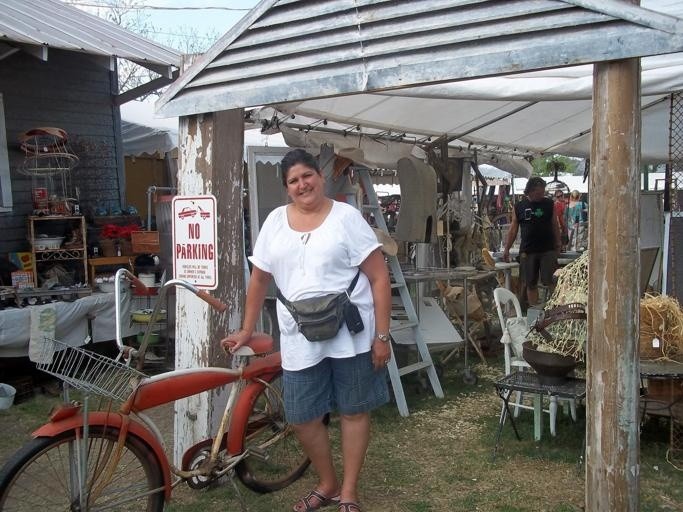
371,228,398,257
524,177,546,197
337,146,377,171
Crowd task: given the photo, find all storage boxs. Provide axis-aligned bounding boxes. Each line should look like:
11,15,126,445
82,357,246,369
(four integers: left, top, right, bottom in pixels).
131,230,160,255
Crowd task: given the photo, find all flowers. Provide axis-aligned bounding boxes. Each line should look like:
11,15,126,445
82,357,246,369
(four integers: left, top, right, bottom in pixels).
98,222,139,239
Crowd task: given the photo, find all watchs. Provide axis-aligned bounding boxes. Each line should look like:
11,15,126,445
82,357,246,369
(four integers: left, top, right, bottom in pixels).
375,332,391,343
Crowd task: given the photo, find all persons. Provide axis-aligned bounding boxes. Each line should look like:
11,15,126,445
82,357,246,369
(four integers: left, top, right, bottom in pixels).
553,189,569,246
561,189,589,251
502,176,562,307
218,148,392,511
500,187,513,213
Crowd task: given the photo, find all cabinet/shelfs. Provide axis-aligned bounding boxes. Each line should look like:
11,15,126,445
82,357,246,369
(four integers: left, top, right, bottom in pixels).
128,287,171,370
31,216,87,290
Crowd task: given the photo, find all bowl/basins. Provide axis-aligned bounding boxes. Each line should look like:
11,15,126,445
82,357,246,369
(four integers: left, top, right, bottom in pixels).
0,382,17,411
130,284,161,296
136,333,161,346
130,311,166,323
26,237,66,251
97,281,133,293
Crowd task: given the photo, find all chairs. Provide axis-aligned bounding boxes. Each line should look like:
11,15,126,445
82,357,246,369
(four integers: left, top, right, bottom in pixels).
434,280,492,368
493,286,577,439
640,375,683,470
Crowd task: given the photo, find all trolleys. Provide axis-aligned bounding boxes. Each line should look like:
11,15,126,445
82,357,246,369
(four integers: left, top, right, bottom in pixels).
415,270,496,394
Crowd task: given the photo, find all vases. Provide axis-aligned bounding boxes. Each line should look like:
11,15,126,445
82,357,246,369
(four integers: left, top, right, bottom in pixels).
99,239,130,257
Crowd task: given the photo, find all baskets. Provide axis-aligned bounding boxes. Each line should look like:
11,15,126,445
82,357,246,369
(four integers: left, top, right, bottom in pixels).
34,335,150,403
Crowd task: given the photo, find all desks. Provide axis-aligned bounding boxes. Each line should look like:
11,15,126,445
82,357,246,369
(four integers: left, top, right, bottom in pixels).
88,256,138,288
385,266,477,394
492,372,589,444
0,282,174,388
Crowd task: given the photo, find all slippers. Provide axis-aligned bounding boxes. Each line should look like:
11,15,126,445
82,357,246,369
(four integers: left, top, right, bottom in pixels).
292,487,362,512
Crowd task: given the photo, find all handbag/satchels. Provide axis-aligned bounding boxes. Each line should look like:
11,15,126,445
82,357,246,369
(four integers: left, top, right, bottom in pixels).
272,267,360,343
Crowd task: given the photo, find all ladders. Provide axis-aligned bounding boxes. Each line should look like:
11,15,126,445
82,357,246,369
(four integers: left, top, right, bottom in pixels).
331,163,445,418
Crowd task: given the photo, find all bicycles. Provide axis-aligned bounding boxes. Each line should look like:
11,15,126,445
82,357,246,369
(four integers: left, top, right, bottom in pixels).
0,258,334,511
